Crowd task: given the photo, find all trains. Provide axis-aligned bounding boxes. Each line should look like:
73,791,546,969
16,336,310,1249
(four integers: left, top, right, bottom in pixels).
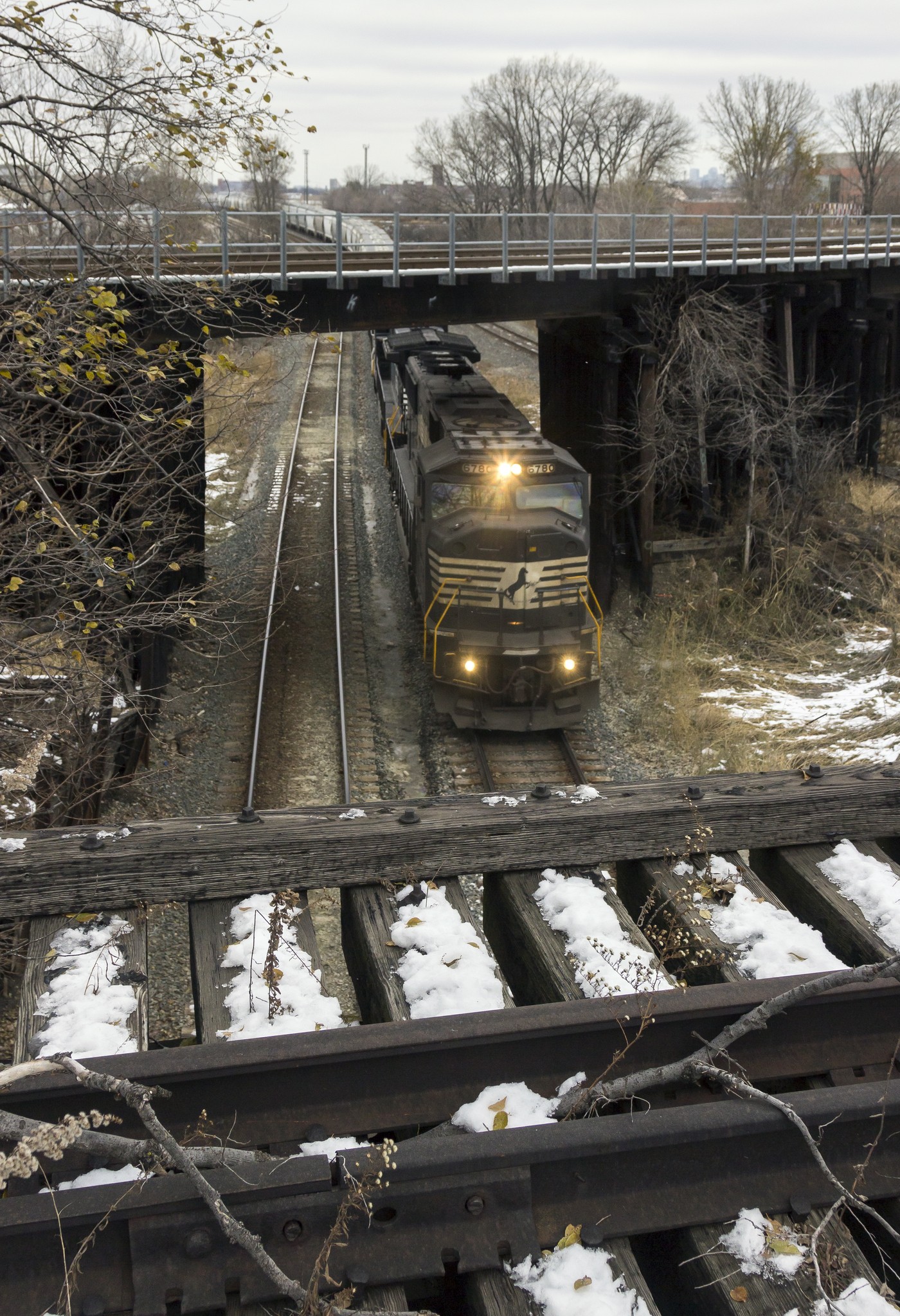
198,188,605,735
